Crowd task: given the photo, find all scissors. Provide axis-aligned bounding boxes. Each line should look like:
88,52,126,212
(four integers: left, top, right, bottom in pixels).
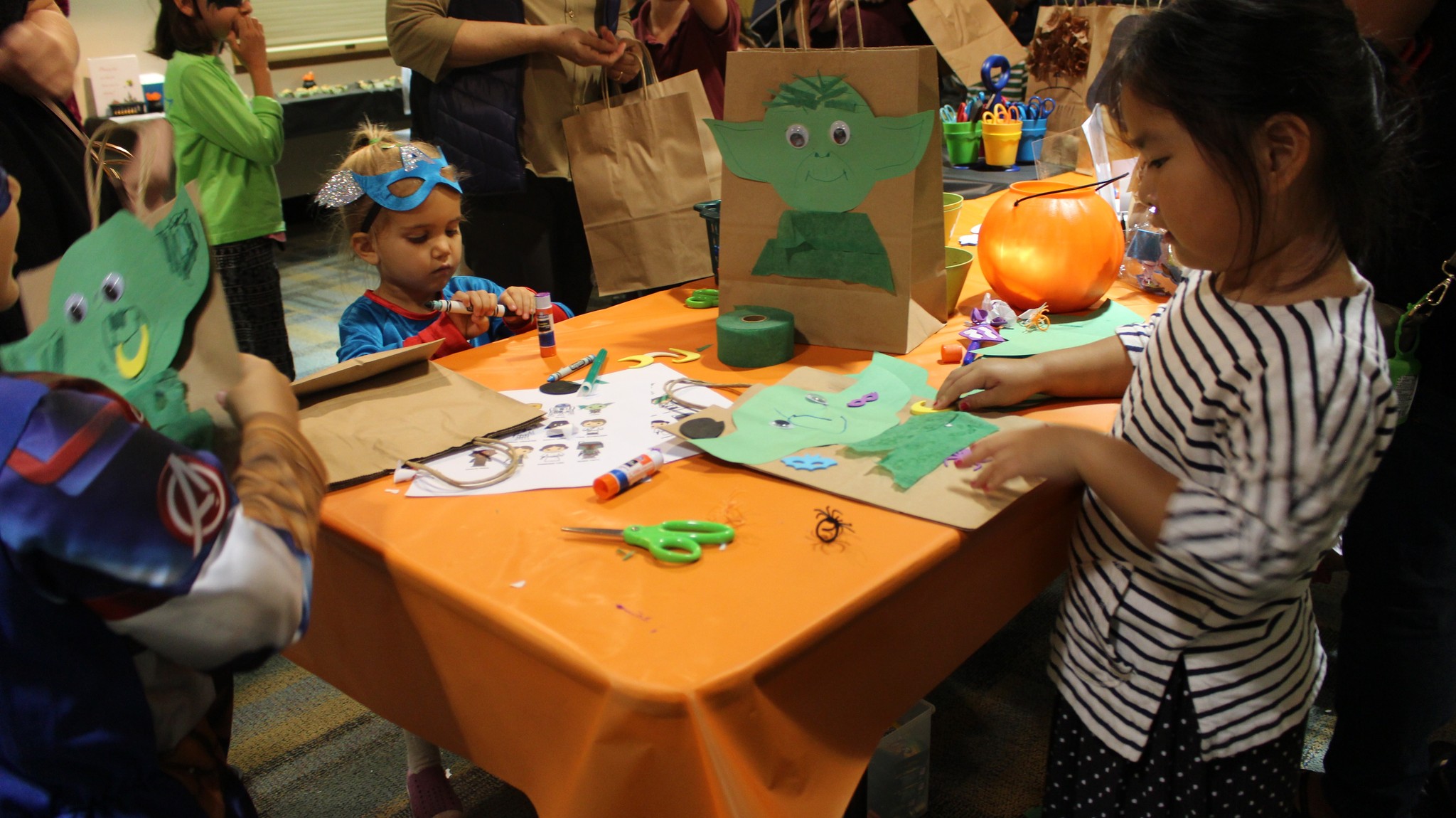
685,289,719,308
938,87,1056,123
559,520,736,564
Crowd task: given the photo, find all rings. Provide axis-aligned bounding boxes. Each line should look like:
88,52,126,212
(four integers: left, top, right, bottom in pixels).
613,71,623,84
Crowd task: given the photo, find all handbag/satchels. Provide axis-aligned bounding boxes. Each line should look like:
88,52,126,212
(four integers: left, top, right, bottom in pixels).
562,37,722,296
1024,0,1167,168
17,120,243,441
717,1,950,355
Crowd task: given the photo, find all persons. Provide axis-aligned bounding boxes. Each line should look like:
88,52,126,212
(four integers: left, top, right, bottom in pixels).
388,0,643,331
0,167,331,818
0,0,84,348
311,118,575,818
629,0,741,118
144,0,296,382
929,0,1401,818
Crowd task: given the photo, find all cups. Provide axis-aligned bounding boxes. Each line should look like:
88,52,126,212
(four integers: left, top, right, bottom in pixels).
1017,118,1048,161
944,246,974,316
943,192,964,246
943,119,982,164
982,119,1023,167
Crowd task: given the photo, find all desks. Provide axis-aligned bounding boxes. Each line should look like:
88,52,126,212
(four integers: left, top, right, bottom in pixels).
278,169,1169,818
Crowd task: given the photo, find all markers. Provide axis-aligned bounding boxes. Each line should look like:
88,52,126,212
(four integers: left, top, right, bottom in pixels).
424,299,520,317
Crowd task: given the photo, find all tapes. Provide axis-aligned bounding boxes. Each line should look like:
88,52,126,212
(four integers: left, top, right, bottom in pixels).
715,305,794,367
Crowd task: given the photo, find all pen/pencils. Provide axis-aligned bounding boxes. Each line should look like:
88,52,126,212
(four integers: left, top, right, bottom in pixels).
957,397,1059,414
964,339,979,364
547,354,596,382
581,348,608,393
